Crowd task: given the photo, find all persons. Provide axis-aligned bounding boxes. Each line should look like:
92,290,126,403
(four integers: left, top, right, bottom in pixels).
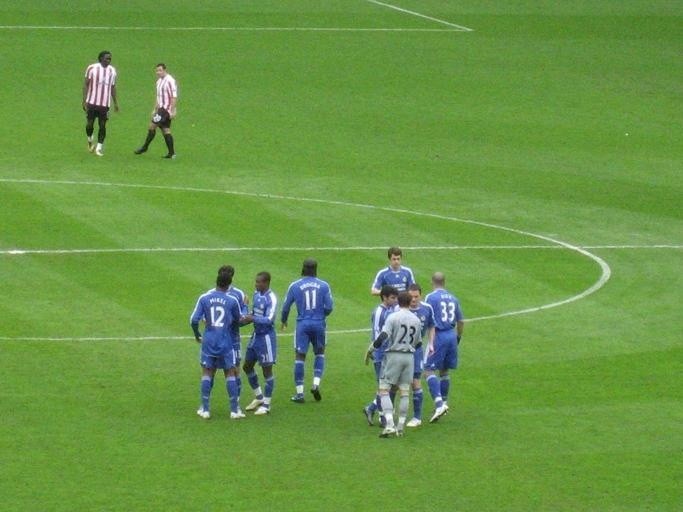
405,282,438,431
81,49,121,157
424,271,463,424
187,272,250,419
362,284,400,427
241,270,281,417
133,62,178,158
363,292,422,438
369,244,416,300
206,263,247,407
281,258,334,404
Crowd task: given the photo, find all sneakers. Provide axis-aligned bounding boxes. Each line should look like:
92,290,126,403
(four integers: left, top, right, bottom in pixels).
290,393,305,403
428,403,450,424
395,426,404,438
363,406,374,426
161,152,176,161
405,417,422,427
253,405,271,416
377,410,386,427
229,409,247,420
311,384,322,402
196,405,211,419
244,396,265,411
134,145,148,155
87,135,105,157
380,427,397,438
